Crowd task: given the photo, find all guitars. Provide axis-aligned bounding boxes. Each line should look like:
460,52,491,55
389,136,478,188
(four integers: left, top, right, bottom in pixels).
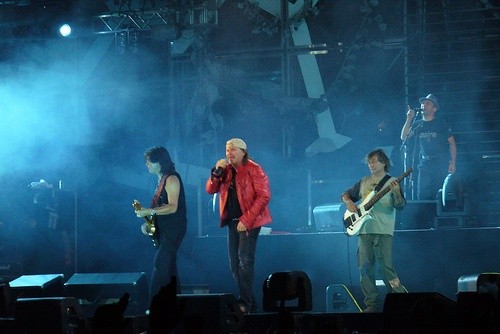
341,168,416,236
132,200,158,245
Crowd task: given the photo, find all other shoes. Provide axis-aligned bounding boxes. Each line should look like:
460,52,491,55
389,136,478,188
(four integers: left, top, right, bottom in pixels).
362,306,380,313
239,303,249,315
145,309,151,316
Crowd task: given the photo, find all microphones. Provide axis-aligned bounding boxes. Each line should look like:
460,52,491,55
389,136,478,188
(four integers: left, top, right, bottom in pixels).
213,158,228,174
415,108,423,111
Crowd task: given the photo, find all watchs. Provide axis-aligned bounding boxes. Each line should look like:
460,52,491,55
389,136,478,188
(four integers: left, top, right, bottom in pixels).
151,207,156,216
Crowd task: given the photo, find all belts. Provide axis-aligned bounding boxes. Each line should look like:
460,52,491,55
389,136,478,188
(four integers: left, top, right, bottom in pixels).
421,154,449,160
228,218,239,221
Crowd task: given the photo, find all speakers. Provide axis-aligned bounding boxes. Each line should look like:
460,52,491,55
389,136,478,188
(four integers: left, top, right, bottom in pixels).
8,274,63,297
175,292,242,316
456,272,500,309
400,200,439,230
64,272,149,315
15,296,86,334
383,292,454,310
313,203,347,233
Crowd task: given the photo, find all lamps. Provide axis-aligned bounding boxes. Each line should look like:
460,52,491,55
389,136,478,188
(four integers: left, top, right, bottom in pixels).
47,23,72,38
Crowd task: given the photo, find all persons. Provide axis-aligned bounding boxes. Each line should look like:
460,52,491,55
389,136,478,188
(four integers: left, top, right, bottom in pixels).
401,94,456,201
135,146,186,297
341,149,407,313
206,137,272,312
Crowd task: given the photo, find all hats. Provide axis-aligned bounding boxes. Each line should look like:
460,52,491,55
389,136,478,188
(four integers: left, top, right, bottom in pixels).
419,94,440,112
225,138,247,150
